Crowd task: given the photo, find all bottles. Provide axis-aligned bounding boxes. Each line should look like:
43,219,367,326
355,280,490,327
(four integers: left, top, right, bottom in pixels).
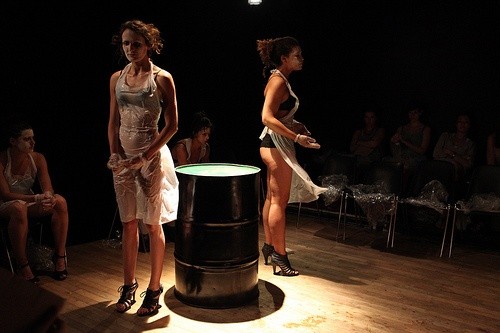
113,230,120,248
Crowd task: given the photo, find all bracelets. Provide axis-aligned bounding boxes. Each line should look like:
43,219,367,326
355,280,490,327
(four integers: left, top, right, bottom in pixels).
139,150,149,162
449,151,456,159
406,141,410,146
294,134,300,143
34,194,39,203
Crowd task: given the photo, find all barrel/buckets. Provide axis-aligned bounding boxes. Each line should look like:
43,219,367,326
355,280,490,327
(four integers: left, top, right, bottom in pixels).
172,160,262,310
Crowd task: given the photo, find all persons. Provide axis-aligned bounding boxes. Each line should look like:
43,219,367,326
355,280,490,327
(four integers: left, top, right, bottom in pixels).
256,37,320,277
106,19,180,317
351,107,500,176
166,112,215,240
0,123,69,288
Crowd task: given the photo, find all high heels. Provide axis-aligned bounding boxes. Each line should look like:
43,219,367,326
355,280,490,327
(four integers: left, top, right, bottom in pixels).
262,243,274,264
17,263,40,286
51,253,68,280
271,251,300,276
137,287,163,317
115,281,138,313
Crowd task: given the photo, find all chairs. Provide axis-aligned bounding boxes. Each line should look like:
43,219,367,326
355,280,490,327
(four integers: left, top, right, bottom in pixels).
0,185,68,275
259,146,500,260
